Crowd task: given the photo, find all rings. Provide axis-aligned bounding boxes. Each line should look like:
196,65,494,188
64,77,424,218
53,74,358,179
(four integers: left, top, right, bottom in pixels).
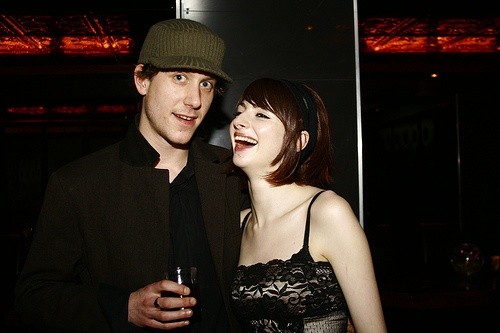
154,297,160,308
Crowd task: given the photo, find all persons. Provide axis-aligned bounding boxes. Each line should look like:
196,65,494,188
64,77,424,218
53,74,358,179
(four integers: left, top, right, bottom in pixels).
229,77,388,333
12,19,250,333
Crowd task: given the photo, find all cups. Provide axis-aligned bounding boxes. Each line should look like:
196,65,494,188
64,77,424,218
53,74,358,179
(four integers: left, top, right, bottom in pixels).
161,266,201,333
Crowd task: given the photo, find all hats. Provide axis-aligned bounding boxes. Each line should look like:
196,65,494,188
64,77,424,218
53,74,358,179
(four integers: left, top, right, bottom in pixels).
138,19,233,83
